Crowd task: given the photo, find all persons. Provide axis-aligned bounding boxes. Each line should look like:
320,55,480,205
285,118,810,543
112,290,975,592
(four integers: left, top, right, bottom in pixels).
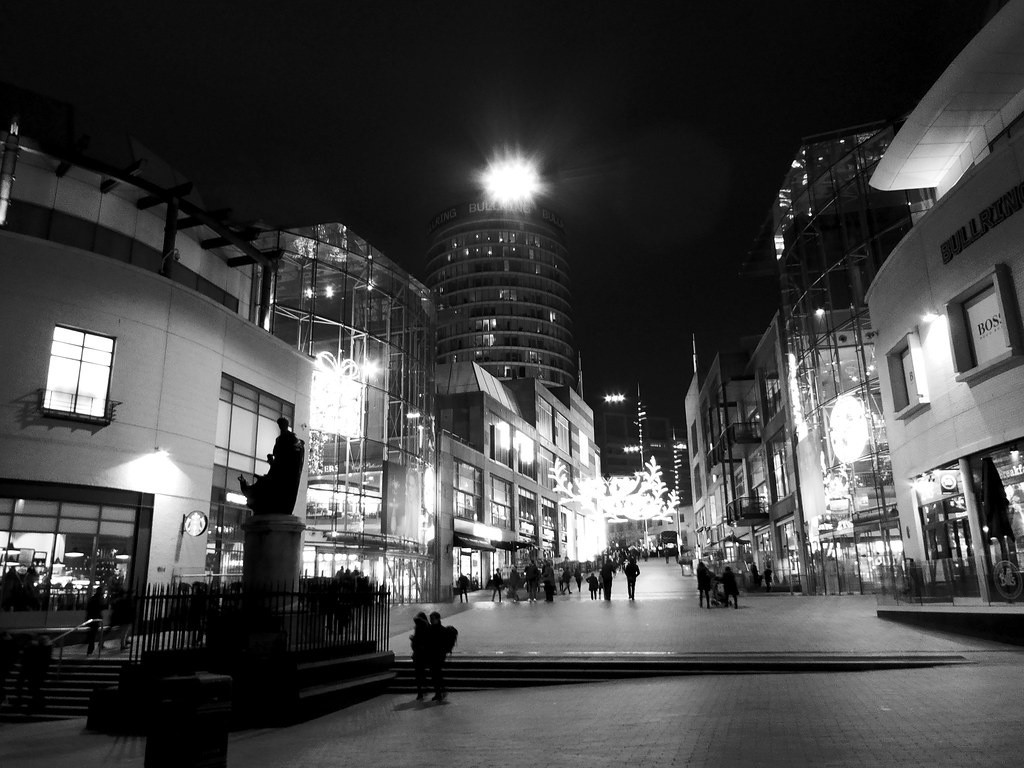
2,566,38,611
558,567,582,594
666,550,669,563
625,558,640,600
509,559,555,602
675,548,679,563
602,559,616,601
764,565,772,592
492,568,504,602
751,565,759,589
408,611,446,704
585,547,640,600
336,566,364,578
720,567,739,609
697,562,712,608
457,573,470,603
238,418,298,497
86,587,135,656
0,631,53,707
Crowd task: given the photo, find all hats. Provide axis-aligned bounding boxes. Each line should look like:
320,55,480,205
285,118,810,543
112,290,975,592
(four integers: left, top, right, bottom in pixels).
413,612,428,625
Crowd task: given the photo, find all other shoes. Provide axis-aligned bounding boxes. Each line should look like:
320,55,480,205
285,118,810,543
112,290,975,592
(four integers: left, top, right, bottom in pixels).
121,646,130,650
126,641,131,644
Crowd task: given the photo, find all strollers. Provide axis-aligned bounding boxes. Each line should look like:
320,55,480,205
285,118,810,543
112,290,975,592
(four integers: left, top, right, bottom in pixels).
711,581,733,607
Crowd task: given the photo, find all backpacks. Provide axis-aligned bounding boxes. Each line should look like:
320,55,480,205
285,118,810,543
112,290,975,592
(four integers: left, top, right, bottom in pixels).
438,625,458,655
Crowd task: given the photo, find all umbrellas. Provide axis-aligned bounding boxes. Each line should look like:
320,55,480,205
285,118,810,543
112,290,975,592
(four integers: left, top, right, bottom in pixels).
980,457,1016,560
